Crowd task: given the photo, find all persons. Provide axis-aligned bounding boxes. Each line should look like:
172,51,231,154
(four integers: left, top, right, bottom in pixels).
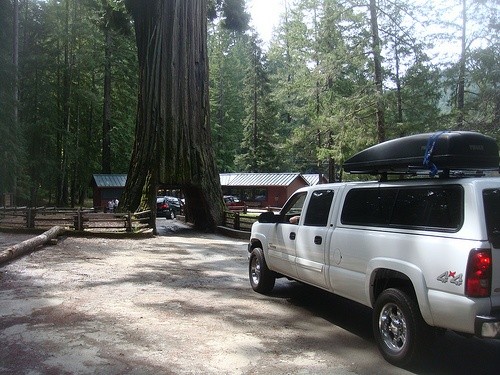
115,197,120,209
108,199,114,212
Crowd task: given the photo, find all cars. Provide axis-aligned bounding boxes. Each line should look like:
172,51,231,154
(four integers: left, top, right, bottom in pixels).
156,196,184,219
223,196,246,213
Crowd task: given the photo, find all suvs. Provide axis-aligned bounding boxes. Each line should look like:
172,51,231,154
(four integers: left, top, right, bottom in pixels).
247,130,500,364
